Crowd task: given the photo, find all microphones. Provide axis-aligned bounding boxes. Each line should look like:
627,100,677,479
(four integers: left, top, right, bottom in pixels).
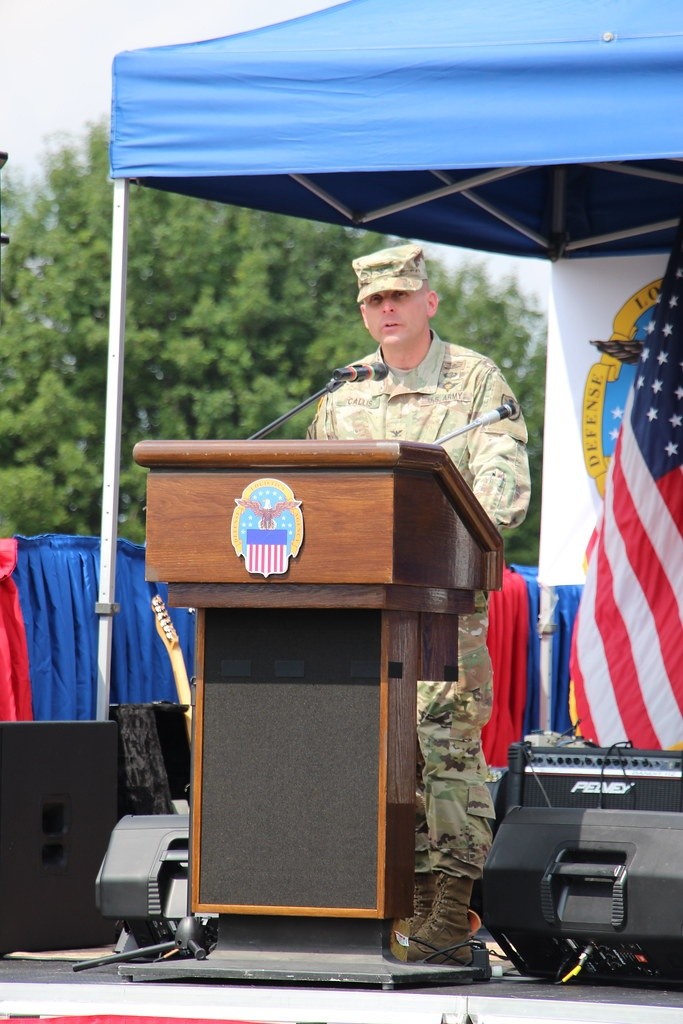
475,403,516,428
333,362,390,382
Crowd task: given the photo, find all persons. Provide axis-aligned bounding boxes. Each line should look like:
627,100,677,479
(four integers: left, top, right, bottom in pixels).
306,245,530,966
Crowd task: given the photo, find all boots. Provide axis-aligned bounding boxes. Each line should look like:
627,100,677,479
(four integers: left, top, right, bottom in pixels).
407,872,474,964
403,872,438,937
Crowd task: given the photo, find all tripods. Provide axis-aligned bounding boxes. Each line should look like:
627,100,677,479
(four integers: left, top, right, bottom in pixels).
71,378,344,973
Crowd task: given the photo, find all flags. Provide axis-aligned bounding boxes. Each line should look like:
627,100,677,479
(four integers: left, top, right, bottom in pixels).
569,219,683,750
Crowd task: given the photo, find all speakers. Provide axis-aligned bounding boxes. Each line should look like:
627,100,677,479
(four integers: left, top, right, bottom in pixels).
481,743,683,993
0,720,122,952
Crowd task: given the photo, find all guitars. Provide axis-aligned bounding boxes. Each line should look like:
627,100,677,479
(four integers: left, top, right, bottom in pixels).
149,592,195,743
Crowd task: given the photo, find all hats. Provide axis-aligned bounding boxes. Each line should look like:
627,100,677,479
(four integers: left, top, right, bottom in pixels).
350,244,429,304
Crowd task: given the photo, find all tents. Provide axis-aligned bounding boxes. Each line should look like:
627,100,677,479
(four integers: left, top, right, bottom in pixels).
96,0,683,718
